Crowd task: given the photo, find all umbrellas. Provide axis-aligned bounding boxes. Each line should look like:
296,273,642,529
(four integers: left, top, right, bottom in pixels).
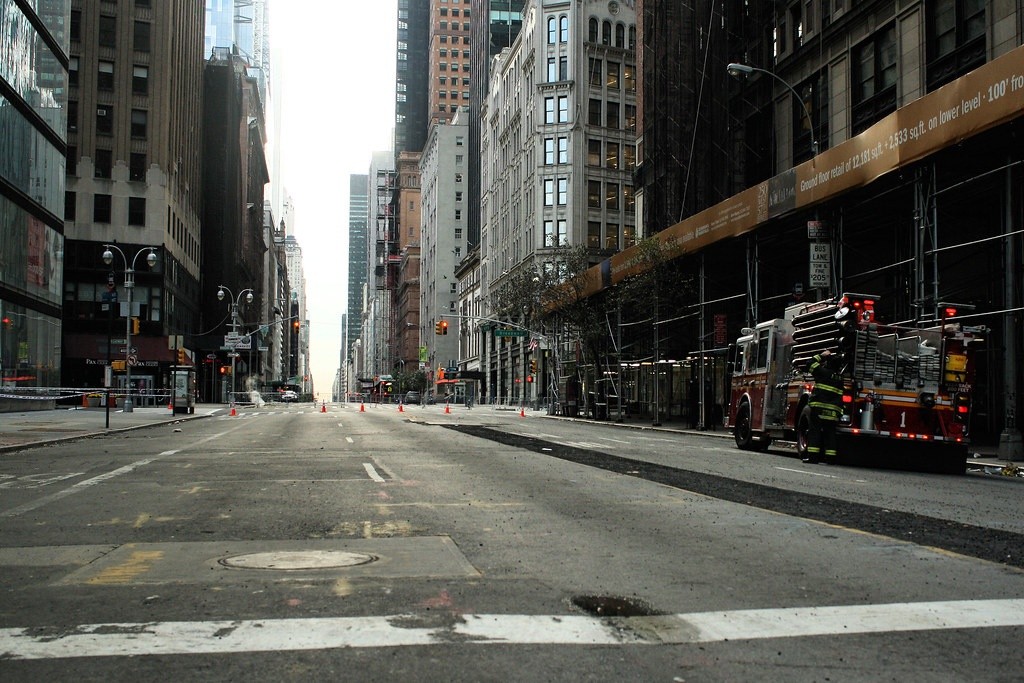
435,379,458,394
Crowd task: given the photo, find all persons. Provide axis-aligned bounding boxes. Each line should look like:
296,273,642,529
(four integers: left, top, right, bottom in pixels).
803,350,849,466
19,336,28,368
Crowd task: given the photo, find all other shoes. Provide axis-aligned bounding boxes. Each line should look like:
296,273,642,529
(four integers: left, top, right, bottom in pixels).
803,458,819,464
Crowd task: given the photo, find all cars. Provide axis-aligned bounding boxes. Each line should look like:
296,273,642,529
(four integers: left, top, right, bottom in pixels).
280,391,299,402
405,391,423,405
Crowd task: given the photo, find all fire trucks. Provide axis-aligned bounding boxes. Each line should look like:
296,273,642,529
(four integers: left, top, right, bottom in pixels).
726,294,977,476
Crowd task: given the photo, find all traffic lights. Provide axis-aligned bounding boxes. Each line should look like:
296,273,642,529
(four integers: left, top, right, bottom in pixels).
443,323,448,335
176,347,186,364
219,366,228,381
436,322,442,335
294,322,300,335
530,358,539,374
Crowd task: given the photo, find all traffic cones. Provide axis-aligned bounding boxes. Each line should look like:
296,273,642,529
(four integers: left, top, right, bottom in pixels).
320,399,327,413
358,399,367,412
229,401,239,416
445,400,451,414
398,400,404,412
520,405,526,418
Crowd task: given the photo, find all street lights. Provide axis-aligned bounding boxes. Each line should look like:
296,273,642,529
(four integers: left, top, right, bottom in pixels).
102,244,158,412
218,284,254,410
407,322,425,407
